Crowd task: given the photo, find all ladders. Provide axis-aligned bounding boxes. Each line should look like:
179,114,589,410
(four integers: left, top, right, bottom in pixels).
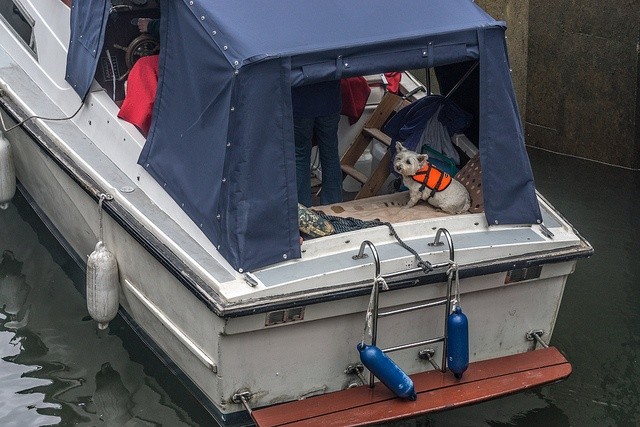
311,91,417,205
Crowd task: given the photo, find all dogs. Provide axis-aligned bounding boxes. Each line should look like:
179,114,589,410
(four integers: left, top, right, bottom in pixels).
392,141,472,216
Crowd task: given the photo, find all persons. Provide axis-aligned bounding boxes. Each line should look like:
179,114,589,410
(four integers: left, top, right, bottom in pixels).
137,17,161,41
291,80,343,208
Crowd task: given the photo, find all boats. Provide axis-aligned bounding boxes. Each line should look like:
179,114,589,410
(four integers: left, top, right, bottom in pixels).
0,0,594,426
